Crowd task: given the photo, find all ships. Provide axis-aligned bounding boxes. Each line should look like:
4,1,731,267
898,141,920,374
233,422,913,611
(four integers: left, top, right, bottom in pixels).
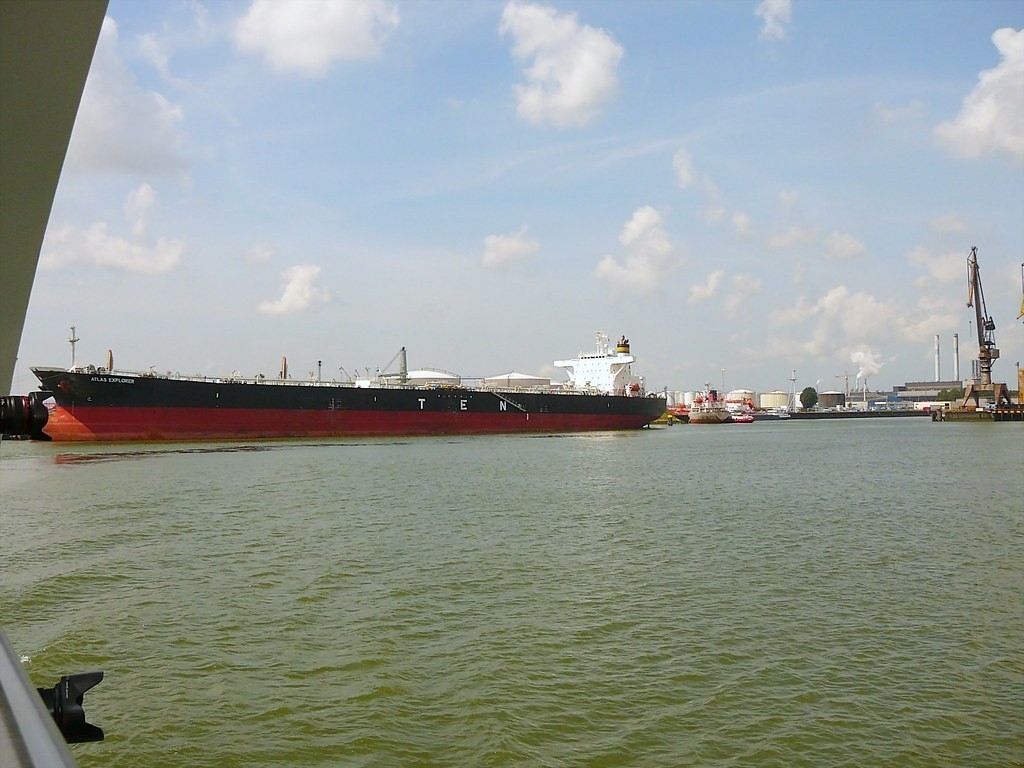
29,326,668,443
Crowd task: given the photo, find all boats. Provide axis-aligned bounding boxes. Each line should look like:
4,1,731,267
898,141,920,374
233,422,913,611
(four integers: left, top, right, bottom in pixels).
666,390,755,423
